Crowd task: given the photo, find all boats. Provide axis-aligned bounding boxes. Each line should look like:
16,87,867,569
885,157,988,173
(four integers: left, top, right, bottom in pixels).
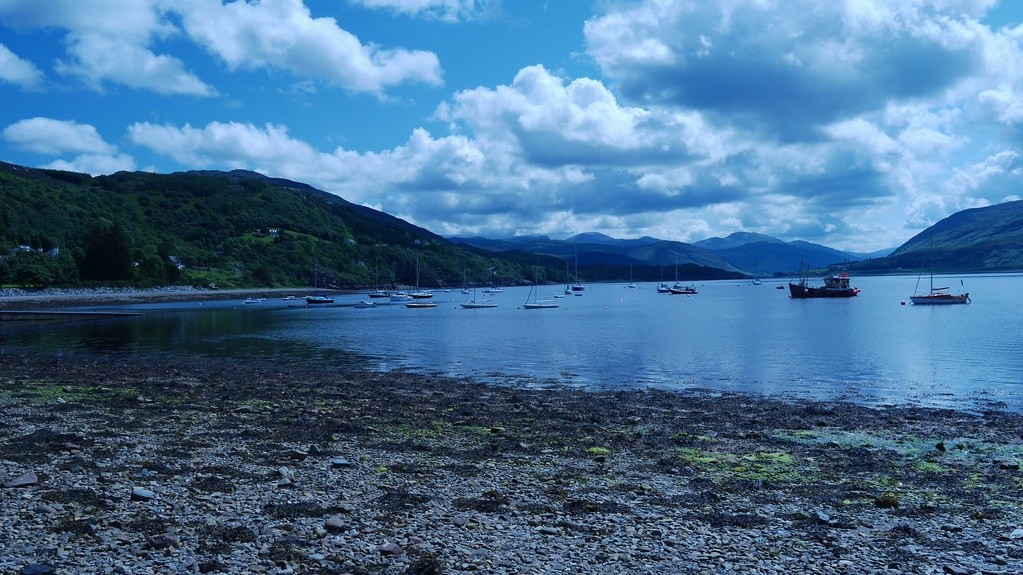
788,260,861,299
353,300,377,309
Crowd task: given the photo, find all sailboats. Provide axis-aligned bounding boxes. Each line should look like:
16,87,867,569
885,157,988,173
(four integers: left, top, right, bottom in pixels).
629,264,637,288
753,264,762,286
367,256,390,298
669,254,699,295
657,262,669,293
305,262,334,305
392,255,504,308
523,257,559,308
909,229,972,305
572,241,585,290
